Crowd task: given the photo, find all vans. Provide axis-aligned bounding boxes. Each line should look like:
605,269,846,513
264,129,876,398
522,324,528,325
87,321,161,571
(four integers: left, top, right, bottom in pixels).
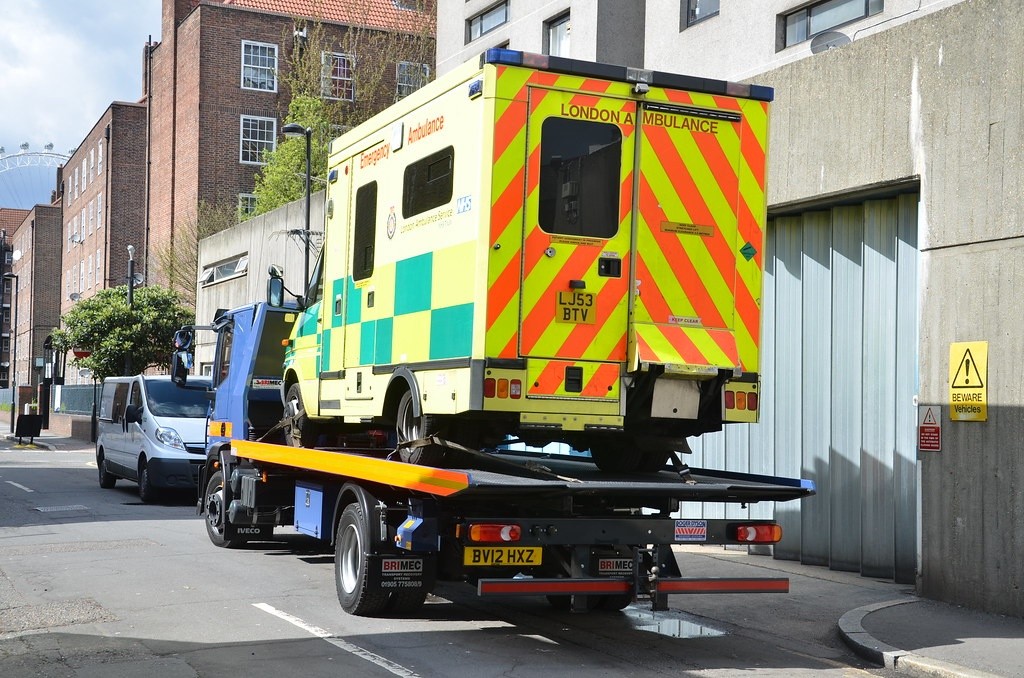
95,374,217,502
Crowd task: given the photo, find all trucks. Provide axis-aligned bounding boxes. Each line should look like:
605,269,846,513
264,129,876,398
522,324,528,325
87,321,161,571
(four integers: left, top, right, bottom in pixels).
170,47,818,616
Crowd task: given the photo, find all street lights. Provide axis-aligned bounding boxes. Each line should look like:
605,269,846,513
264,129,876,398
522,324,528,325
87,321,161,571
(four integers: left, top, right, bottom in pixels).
4,272,18,434
281,122,312,299
